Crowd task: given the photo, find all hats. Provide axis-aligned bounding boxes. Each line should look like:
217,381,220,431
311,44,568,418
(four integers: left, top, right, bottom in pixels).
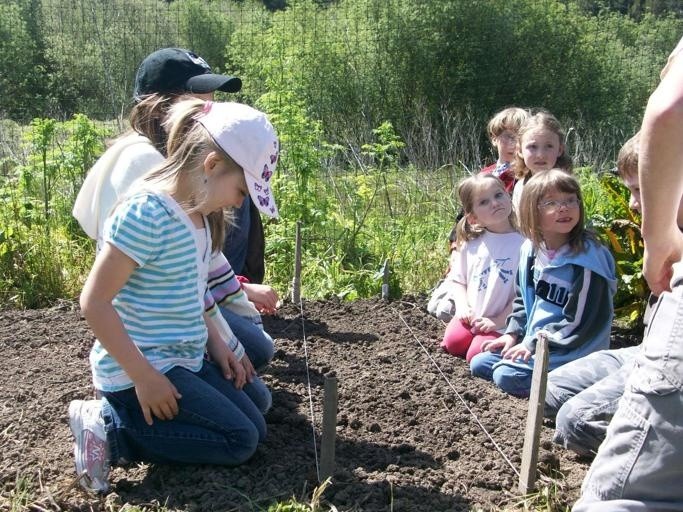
192,100,281,219
132,48,242,95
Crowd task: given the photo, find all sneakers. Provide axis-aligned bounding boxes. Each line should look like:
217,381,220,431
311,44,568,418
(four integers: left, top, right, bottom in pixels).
67,400,109,497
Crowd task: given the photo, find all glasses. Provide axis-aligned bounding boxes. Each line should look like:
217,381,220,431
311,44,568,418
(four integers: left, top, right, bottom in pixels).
537,197,582,210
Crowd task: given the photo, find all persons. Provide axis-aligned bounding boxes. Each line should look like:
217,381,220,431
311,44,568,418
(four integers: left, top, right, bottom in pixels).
512,109,574,237
543,123,683,457
570,32,683,511
470,168,616,397
429,106,536,323
62,44,281,493
439,170,531,363
